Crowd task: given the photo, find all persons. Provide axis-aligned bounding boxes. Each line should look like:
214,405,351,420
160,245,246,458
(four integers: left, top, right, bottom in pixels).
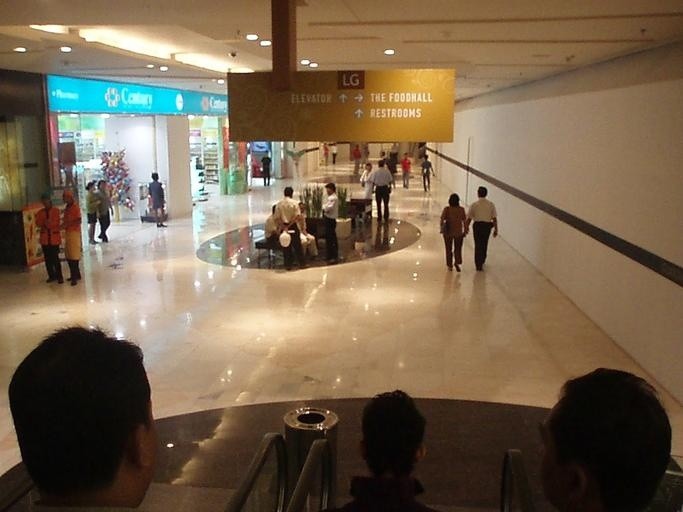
538,367,672,511
466,186,497,270
149,172,167,227
8,323,156,512
440,193,468,272
266,143,436,269
35,180,113,285
318,390,439,512
261,150,271,186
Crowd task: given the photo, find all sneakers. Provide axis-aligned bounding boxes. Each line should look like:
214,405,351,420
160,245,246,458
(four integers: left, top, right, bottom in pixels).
57,275,63,284
475,261,483,271
66,276,81,286
88,234,108,245
46,276,56,282
449,263,461,272
156,222,168,228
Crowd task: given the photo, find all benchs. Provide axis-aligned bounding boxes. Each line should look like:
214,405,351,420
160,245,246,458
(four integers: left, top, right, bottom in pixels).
255,238,276,269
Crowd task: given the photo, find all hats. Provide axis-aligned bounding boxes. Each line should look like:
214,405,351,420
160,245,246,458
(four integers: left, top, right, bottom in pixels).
279,231,293,249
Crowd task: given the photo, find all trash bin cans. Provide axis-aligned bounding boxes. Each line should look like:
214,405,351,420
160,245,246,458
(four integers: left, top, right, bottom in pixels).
229,169,246,194
284,407,339,510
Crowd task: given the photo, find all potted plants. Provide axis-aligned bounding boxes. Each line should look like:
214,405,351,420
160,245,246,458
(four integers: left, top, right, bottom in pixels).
302,182,325,238
334,187,352,240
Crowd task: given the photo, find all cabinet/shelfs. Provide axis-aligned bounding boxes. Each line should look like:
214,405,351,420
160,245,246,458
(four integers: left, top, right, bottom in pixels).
204,152,219,184
